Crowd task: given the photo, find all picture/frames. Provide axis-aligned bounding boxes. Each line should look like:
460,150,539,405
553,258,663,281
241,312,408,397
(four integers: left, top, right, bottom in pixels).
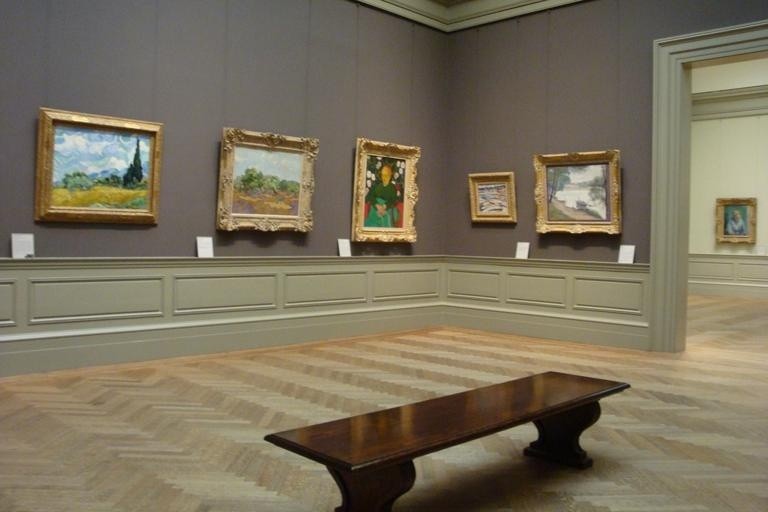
34,106,163,227
533,151,622,234
715,197,756,245
351,137,421,244
216,128,319,232
468,171,517,223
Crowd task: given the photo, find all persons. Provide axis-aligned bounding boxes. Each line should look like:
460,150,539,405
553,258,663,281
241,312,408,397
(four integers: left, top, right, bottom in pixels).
726,210,746,235
365,162,399,228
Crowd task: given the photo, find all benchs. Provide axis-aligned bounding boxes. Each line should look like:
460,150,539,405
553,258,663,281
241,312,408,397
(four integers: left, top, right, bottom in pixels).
263,370,630,512
